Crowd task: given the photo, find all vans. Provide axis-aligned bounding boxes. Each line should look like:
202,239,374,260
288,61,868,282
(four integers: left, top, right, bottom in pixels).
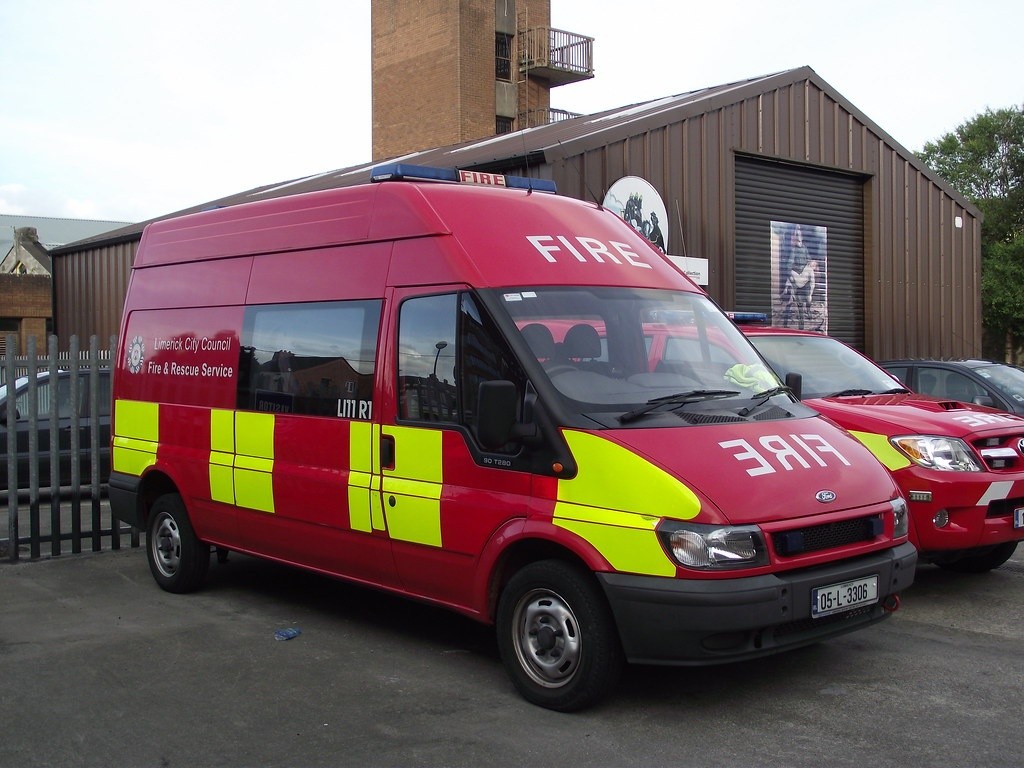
109,161,922,715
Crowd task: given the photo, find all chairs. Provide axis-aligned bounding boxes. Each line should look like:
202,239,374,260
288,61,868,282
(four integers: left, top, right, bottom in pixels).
522,321,610,376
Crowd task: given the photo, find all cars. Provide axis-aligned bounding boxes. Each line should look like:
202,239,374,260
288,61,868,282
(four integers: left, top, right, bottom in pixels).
876,354,1024,417
0,367,110,504
508,309,1024,577
